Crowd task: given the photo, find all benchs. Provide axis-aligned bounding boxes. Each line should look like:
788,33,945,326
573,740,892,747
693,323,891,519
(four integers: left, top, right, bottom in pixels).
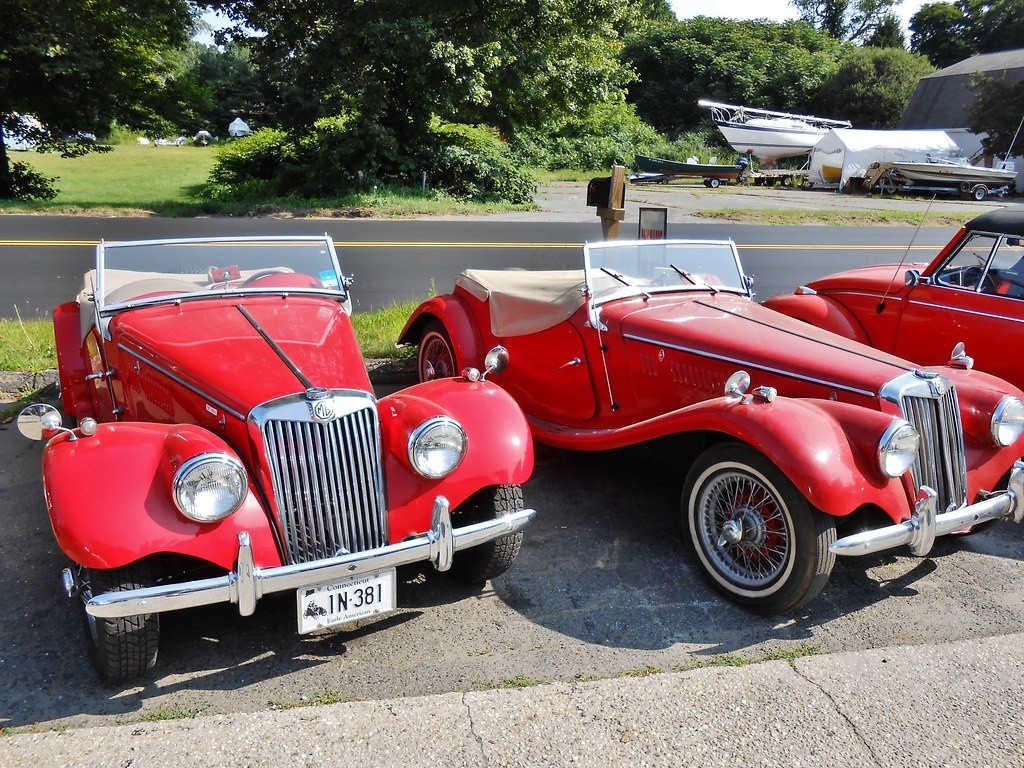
84,267,296,301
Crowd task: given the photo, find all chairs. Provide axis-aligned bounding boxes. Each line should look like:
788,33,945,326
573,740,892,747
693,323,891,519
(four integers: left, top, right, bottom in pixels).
243,272,323,289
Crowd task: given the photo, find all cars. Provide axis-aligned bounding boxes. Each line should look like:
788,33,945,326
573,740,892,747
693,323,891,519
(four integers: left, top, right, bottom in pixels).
57,129,97,143
758,208,1024,393
15,233,536,684
394,237,1024,621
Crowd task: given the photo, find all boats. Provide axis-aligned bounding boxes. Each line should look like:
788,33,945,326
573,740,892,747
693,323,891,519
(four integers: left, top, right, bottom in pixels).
712,117,830,160
634,153,748,180
135,129,219,148
228,117,254,138
889,160,1018,183
